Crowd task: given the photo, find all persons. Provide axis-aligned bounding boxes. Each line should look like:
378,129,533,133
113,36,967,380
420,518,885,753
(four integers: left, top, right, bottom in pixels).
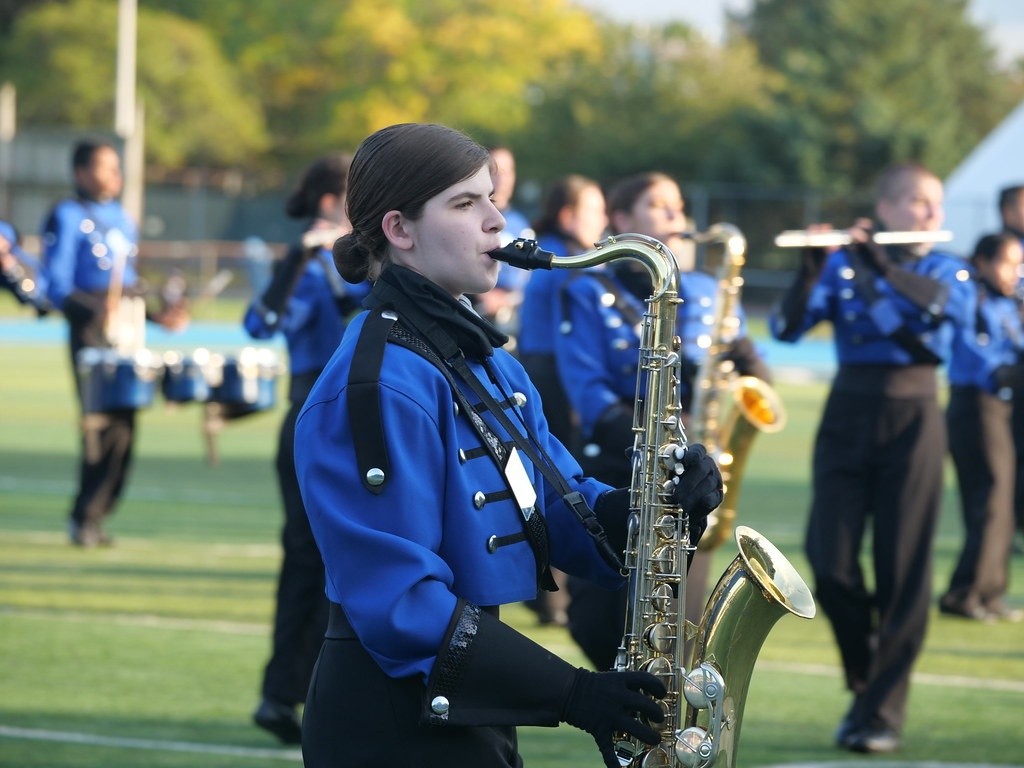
290,121,722,767
0,138,1024,754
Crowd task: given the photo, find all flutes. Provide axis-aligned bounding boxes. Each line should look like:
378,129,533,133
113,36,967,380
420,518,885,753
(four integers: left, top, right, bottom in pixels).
773,215,955,251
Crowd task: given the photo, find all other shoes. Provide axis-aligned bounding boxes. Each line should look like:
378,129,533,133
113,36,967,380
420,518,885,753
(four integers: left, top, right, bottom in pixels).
939,593,1023,624
254,698,302,745
835,705,904,752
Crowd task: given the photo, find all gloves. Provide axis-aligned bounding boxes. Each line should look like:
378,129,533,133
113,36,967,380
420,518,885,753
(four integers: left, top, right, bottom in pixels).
417,596,669,768
593,444,724,573
261,239,323,317
590,402,643,453
722,336,772,380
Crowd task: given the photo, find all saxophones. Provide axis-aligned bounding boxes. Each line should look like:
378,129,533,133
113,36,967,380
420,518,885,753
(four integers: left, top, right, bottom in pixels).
671,221,791,554
485,232,817,768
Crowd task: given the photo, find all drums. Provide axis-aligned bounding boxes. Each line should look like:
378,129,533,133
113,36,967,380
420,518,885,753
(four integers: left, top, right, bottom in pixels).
87,350,277,421
